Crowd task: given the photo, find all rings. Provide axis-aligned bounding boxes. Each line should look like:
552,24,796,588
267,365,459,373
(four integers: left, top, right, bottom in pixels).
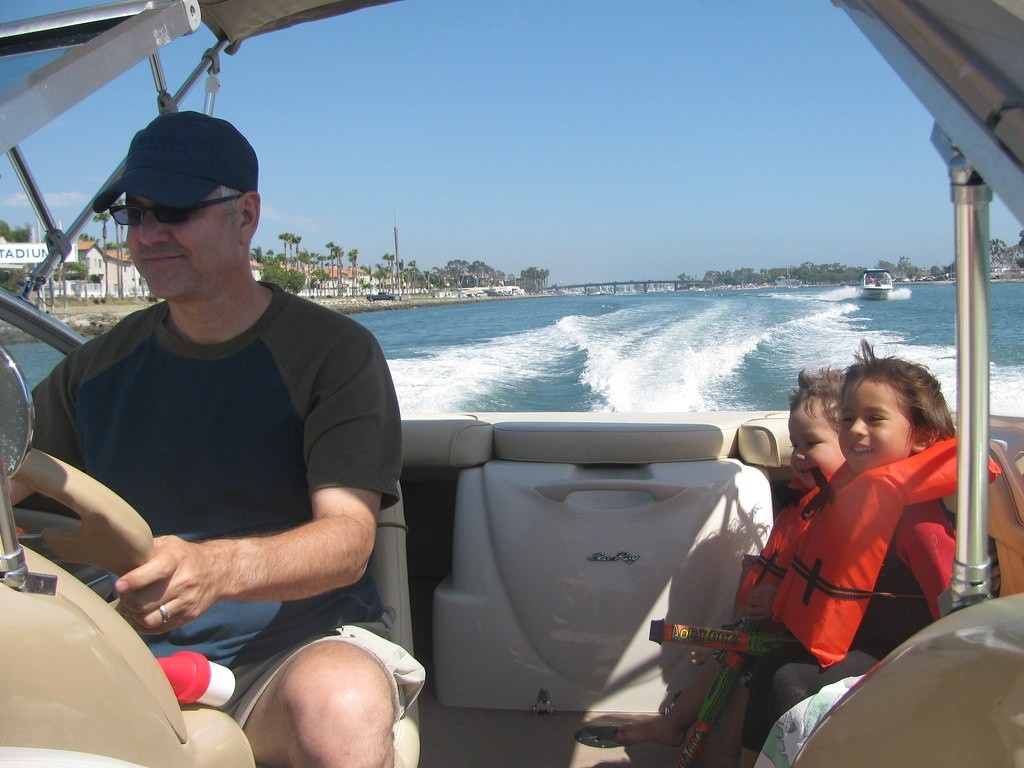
158,604,168,624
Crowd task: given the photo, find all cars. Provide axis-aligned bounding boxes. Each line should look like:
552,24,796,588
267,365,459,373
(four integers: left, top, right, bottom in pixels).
893,275,946,282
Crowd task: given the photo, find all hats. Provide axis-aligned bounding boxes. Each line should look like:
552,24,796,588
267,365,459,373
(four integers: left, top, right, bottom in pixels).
92,112,259,214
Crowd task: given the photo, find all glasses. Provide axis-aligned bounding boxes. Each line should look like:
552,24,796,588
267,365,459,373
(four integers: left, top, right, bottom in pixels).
108,194,244,225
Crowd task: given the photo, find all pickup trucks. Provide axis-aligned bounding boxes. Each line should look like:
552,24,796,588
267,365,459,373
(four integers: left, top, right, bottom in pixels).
367,292,395,302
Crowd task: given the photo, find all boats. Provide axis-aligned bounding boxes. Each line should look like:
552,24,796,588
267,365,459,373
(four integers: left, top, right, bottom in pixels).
862,268,893,301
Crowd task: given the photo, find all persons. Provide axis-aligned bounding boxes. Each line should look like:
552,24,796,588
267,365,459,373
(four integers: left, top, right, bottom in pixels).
866,274,875,284
0,110,425,768
617,342,1001,768
